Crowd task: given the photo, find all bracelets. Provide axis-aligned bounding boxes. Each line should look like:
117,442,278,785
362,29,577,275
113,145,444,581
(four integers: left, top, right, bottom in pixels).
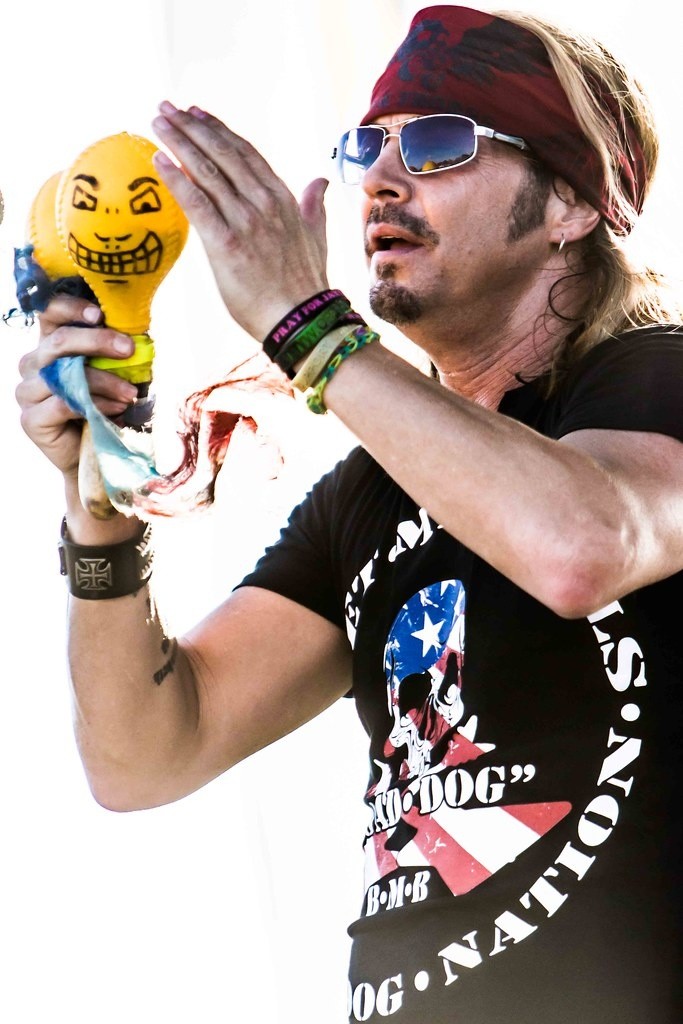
261,290,384,414
58,516,153,602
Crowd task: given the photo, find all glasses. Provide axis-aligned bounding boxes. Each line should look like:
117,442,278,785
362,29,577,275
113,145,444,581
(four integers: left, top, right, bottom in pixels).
332,114,533,186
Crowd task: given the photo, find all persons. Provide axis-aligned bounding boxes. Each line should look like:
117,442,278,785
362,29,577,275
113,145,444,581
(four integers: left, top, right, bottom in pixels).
13,1,683,1024
